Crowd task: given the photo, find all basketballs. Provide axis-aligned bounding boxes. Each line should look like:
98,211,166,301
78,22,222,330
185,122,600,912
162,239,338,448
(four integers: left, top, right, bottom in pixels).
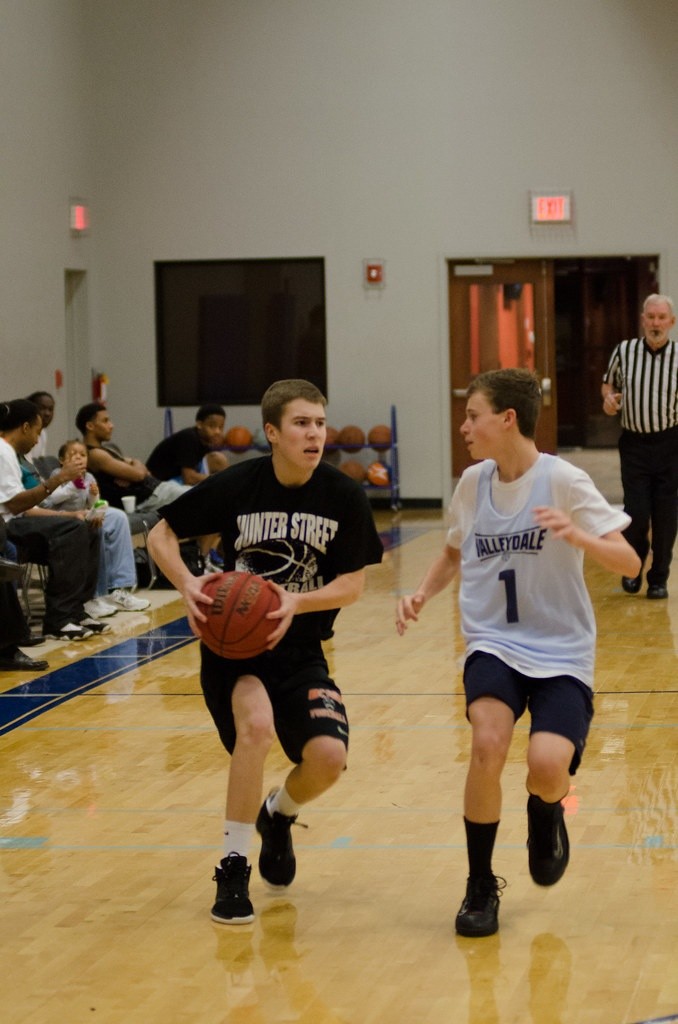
192,570,282,660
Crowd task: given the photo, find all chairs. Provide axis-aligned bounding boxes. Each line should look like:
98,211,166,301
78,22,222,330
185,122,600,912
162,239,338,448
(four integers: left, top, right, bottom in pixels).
15,457,160,637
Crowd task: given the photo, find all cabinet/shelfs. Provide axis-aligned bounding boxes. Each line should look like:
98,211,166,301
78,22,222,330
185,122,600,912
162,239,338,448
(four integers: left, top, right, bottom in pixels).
164,403,402,531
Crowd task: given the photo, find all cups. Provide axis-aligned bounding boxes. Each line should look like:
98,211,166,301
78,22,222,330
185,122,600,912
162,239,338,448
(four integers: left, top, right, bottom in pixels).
121,496,135,513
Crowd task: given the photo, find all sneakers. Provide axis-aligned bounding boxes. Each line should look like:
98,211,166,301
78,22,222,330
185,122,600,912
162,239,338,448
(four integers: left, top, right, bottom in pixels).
527,798,569,886
101,589,151,611
83,599,118,620
256,788,308,890
455,873,507,937
42,622,94,640
211,852,255,925
73,618,111,634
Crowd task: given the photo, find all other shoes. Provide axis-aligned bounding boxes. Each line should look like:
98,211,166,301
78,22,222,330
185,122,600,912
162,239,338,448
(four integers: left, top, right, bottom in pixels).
199,549,224,573
17,635,46,647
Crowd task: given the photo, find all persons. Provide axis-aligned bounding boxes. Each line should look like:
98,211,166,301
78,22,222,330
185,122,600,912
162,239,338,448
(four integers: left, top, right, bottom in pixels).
0,391,229,672
395,368,642,937
146,379,385,924
601,294,678,599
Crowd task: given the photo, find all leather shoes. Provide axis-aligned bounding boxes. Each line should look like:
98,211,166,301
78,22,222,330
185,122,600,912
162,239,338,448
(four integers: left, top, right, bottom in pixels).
0,650,49,671
0,556,25,583
622,541,650,593
647,584,668,599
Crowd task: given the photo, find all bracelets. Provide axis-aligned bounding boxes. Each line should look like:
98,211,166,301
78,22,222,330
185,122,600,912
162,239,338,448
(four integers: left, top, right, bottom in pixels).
42,482,52,494
